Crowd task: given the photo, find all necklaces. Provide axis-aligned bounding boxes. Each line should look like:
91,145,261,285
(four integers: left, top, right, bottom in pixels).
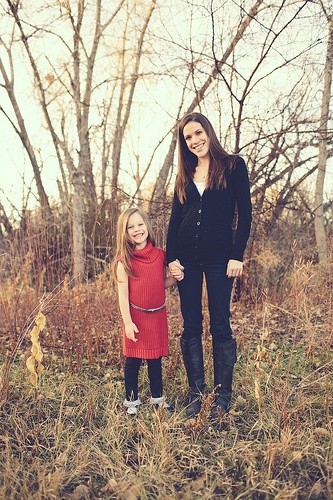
196,165,208,178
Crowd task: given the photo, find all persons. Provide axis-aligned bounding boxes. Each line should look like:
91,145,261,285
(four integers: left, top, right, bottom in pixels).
112,207,184,419
167,112,253,416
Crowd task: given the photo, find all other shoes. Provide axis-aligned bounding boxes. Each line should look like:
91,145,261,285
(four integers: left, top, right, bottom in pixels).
122,399,140,420
149,394,176,412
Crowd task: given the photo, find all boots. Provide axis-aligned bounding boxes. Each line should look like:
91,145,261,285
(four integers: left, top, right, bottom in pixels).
211,338,237,423
180,334,206,417
